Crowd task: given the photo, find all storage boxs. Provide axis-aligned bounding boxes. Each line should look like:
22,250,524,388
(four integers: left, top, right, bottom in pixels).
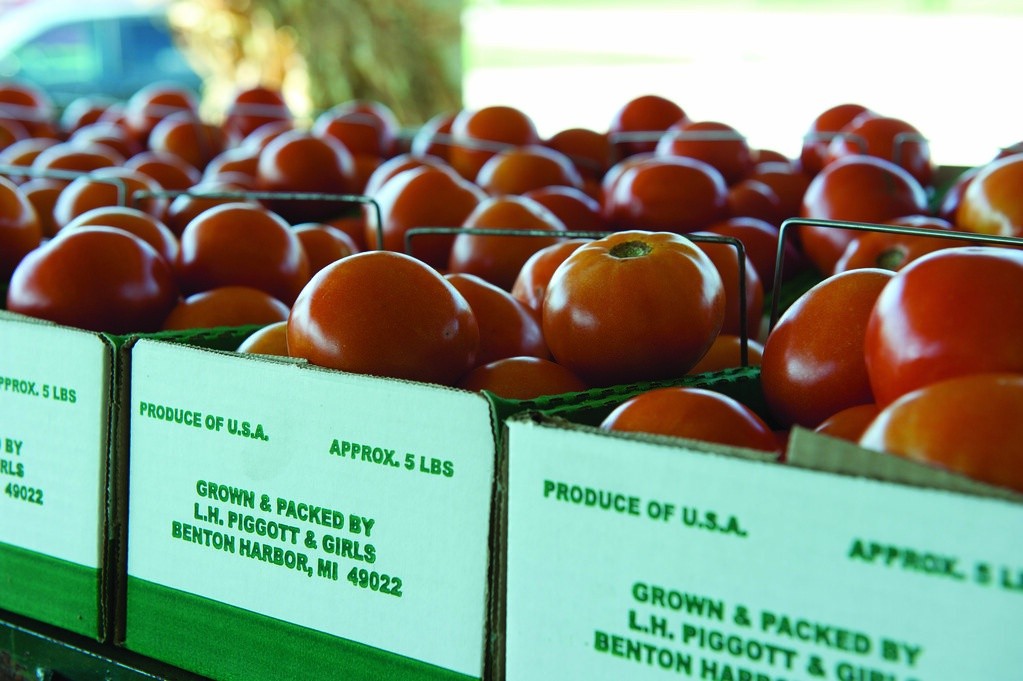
1,309,1023,681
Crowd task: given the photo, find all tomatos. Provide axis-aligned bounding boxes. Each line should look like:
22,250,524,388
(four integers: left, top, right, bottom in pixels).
0,77,1023,492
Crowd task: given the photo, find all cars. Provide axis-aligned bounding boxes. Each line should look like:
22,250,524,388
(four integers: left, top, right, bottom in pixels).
0,4,207,102
